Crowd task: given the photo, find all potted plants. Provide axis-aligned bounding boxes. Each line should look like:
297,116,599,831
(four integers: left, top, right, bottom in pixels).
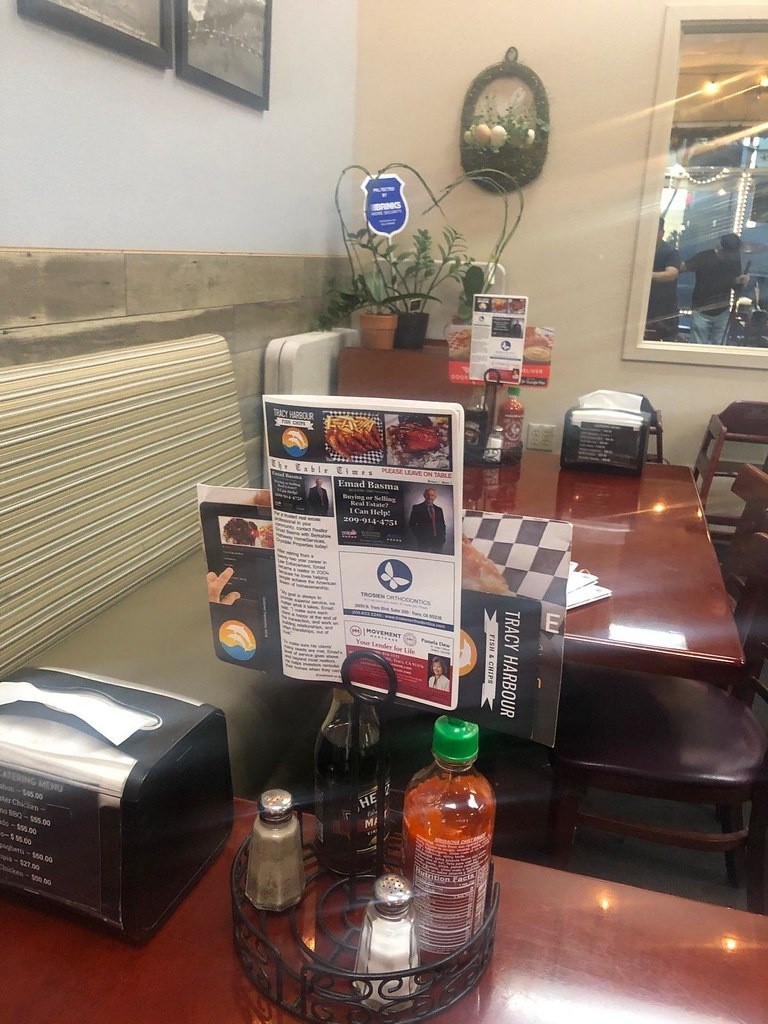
452,264,491,325
318,226,474,351
359,260,399,352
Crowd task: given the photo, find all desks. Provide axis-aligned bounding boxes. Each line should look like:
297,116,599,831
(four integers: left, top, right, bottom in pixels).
456,450,744,685
334,340,477,413
1,799,768,1023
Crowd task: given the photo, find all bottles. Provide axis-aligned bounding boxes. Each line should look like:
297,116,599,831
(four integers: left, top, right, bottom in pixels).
497,386,525,456
313,687,391,878
463,384,490,460
401,715,495,969
350,873,420,1014
242,788,306,911
482,424,505,463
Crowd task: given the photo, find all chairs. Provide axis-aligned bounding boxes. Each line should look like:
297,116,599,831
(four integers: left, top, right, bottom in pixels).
691,414,728,512
719,461,768,643
545,531,768,919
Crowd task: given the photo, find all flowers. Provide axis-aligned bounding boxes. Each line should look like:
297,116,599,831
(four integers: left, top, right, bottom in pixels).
463,97,549,153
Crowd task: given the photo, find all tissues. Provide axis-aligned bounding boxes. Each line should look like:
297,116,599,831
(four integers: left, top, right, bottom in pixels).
560,390,651,477
0,663,236,950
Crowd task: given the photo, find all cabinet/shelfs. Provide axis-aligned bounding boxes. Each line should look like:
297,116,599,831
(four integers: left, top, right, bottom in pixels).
701,401,767,539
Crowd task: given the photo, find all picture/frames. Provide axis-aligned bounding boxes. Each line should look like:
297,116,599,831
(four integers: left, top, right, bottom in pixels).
17,1,174,72
173,1,274,110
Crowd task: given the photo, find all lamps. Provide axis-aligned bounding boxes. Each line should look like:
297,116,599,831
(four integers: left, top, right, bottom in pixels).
701,69,768,101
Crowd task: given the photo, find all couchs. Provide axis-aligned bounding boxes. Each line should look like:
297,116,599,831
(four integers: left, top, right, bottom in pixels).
1,334,324,798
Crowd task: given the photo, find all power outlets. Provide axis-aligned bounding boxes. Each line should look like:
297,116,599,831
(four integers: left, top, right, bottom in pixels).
528,423,557,452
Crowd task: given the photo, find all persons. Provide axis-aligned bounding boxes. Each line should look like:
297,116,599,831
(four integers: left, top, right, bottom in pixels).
645,215,683,341
510,318,522,337
303,473,332,516
680,233,751,345
402,485,450,553
